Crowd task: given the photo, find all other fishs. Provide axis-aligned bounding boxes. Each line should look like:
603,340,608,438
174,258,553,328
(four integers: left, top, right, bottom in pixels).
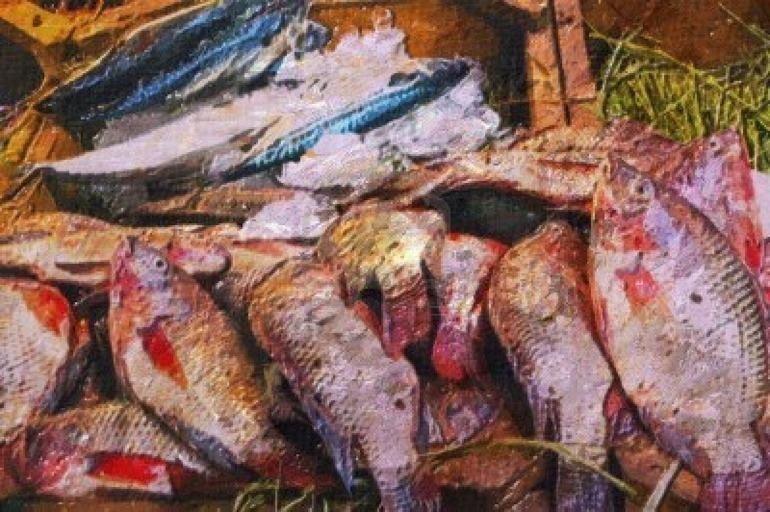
0,0,770,512
